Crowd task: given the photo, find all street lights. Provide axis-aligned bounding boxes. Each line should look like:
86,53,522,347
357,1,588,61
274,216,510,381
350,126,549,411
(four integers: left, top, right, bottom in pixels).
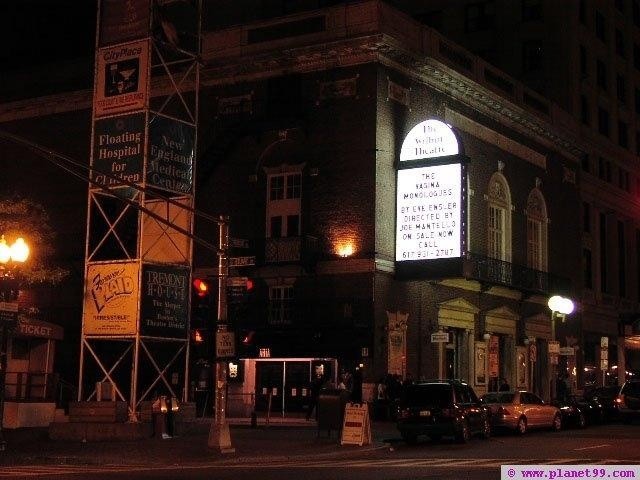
0,232,31,329
548,295,576,395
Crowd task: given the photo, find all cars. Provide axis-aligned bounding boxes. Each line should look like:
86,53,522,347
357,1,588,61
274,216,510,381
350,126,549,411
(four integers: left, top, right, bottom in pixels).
554,392,602,429
481,390,562,435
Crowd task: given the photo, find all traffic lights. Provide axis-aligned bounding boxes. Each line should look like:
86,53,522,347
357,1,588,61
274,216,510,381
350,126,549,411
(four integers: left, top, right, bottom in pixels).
195,277,211,295
241,328,254,344
191,330,206,342
244,278,256,295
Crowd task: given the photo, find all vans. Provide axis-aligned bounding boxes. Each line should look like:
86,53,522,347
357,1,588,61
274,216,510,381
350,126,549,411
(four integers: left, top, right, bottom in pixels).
396,378,490,445
615,379,640,418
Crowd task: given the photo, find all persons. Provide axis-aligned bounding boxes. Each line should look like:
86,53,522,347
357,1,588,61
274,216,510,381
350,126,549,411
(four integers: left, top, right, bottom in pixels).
377,371,416,402
302,372,349,421
